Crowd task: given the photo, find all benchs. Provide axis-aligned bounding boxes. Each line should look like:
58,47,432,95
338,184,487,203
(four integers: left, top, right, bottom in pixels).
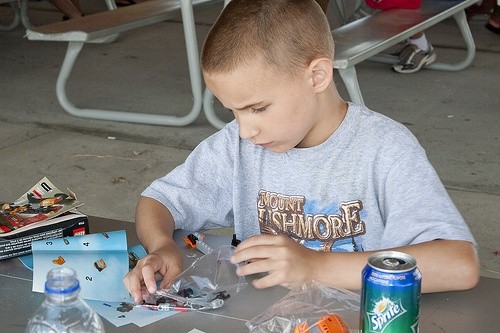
331,0,485,107
26,1,215,126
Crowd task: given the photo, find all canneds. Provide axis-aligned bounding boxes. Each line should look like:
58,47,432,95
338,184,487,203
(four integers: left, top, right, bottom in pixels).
359,251,421,333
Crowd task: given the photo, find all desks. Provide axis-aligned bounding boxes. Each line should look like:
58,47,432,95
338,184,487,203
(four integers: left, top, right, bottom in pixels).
0,207,500,333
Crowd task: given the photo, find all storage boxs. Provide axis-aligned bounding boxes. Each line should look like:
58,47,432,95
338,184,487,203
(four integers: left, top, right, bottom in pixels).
0,178,97,259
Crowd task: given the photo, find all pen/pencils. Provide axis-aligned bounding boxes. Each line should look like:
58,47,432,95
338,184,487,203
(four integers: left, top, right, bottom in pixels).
134,304,197,311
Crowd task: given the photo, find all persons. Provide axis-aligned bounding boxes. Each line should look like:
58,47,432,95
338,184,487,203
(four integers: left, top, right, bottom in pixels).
391,0,500,73
122,0,480,305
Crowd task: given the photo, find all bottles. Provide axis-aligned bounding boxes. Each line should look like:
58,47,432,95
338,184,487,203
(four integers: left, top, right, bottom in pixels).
24,267,106,333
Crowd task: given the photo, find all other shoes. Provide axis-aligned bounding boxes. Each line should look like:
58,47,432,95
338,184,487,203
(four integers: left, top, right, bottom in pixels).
485,11,500,33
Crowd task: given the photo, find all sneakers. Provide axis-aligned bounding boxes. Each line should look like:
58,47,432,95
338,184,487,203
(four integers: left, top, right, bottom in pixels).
391,41,436,74
